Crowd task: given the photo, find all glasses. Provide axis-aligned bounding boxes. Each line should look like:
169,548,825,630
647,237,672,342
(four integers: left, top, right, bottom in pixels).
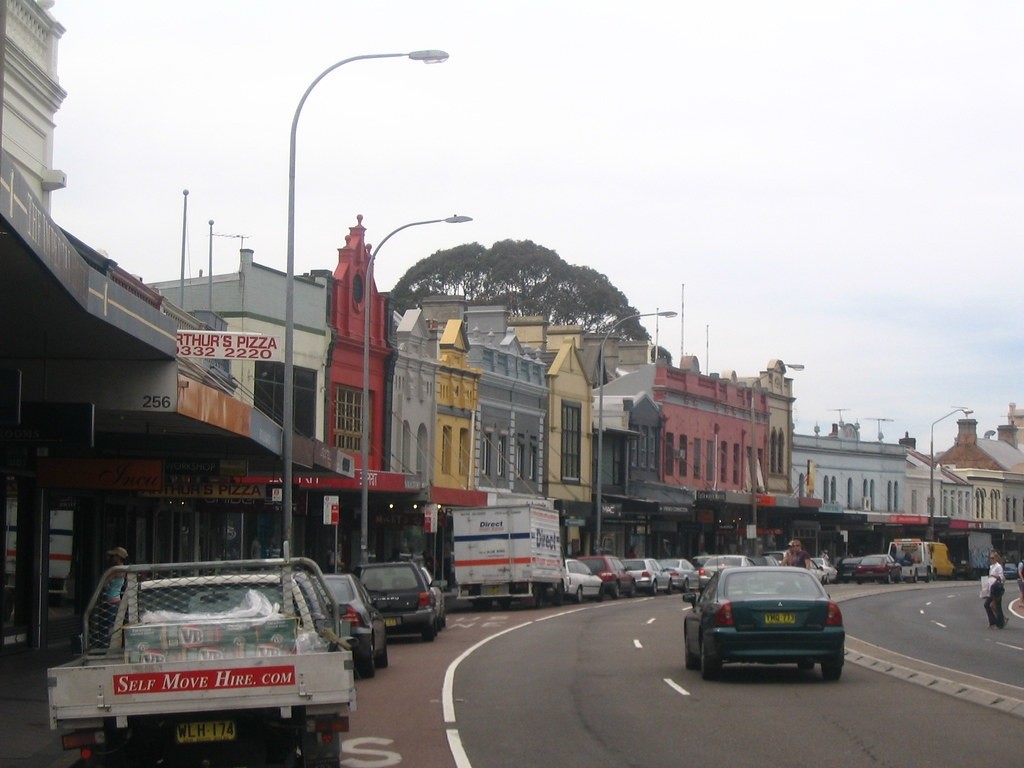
793,545,800,548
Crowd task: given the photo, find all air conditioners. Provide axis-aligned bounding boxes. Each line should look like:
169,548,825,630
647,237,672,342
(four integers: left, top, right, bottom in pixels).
674,448,685,459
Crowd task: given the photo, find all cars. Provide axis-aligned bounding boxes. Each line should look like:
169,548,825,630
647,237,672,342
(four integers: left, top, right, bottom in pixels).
656,558,700,591
307,576,390,678
360,560,446,640
691,552,842,585
683,568,846,681
578,557,636,599
1006,563,1021,580
620,558,674,595
840,554,902,584
561,559,606,602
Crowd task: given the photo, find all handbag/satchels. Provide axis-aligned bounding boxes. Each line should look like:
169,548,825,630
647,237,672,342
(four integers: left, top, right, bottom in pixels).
990,579,1005,596
978,575,997,598
1018,582,1024,592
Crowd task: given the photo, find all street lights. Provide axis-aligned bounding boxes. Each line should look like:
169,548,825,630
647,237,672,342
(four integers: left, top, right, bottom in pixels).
929,409,973,540
597,310,677,558
361,215,474,564
283,49,448,618
752,364,805,555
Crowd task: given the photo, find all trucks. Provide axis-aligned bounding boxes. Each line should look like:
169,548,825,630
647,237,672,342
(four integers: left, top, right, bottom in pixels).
938,533,999,581
47,558,355,768
450,504,568,606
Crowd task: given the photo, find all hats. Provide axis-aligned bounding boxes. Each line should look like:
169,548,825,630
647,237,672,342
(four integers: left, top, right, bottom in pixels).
107,547,128,559
788,540,794,546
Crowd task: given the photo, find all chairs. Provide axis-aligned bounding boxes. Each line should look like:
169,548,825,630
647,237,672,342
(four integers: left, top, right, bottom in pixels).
392,577,414,589
367,576,383,591
775,579,799,594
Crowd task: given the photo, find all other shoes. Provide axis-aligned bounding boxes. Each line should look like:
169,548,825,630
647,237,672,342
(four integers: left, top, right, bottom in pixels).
1002,616,1009,625
986,623,1000,630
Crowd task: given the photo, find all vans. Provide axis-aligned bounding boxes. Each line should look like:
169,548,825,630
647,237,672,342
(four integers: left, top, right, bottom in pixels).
889,541,958,581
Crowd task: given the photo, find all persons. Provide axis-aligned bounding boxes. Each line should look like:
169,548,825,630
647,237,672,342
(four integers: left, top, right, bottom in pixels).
326,543,345,573
428,556,441,580
782,540,810,569
984,552,1009,629
1017,556,1024,606
627,546,636,558
250,532,280,559
96,548,128,656
819,549,829,564
736,543,741,554
